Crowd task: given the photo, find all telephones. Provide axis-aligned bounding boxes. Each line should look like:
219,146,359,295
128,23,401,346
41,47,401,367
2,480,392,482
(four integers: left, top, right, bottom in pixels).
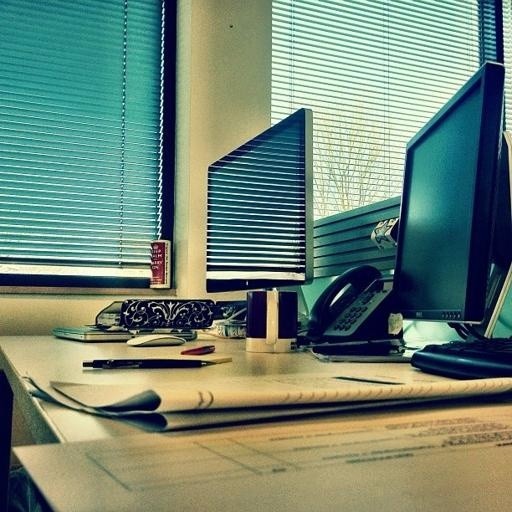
307,265,393,354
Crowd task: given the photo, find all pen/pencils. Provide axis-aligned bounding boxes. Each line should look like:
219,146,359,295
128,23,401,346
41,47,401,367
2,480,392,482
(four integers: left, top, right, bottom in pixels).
82,356,220,371
180,345,215,356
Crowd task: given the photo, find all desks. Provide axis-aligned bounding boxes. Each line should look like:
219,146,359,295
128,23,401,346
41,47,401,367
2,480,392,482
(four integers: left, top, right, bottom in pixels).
0,334,459,512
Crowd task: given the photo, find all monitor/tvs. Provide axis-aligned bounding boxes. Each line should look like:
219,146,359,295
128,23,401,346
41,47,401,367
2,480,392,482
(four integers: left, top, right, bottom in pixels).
206,109,314,352
391,62,511,338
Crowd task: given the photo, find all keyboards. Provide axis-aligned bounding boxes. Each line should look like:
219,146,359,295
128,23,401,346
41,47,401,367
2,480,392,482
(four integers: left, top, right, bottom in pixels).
411,338,512,379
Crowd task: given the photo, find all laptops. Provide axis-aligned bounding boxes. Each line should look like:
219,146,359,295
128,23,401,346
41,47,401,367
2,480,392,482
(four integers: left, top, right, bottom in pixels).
55,327,198,341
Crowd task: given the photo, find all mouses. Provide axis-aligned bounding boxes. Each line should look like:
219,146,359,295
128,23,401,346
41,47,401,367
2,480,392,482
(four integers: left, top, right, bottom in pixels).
127,334,186,346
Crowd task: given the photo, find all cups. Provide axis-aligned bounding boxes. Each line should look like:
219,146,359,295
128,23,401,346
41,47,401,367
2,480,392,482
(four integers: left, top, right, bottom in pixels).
245,289,300,354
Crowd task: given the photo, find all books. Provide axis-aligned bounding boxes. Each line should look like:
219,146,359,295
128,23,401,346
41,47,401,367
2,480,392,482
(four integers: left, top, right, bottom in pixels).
54,324,194,342
